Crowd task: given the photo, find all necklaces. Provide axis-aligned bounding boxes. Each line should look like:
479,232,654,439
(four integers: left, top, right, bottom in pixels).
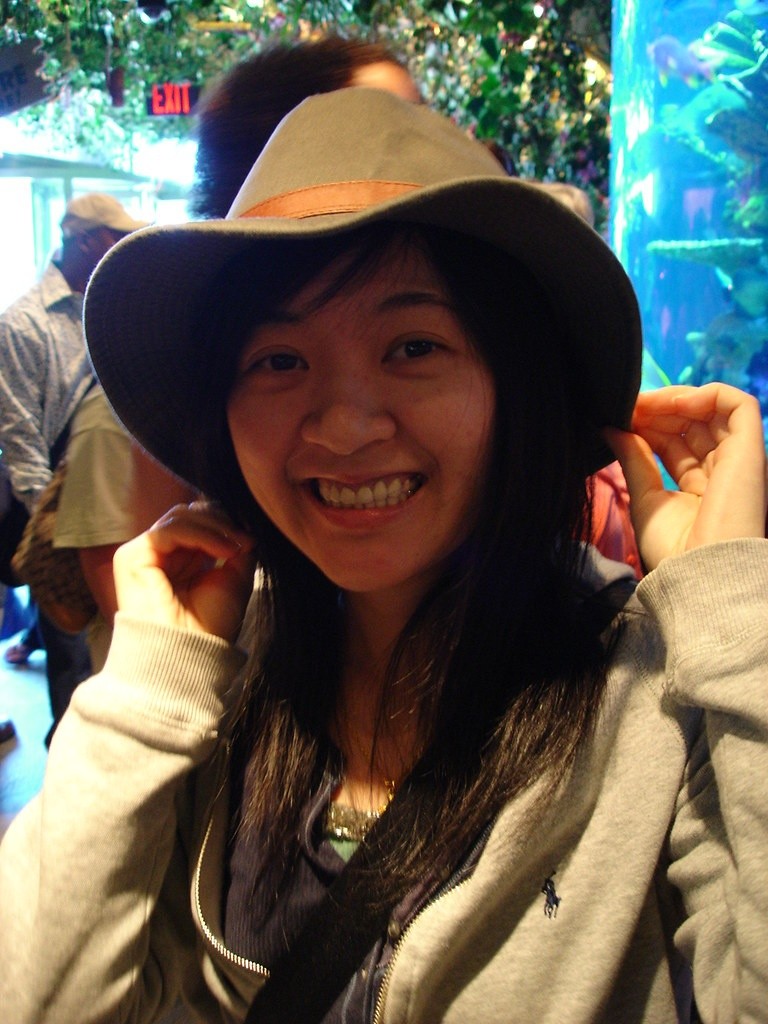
338,708,397,802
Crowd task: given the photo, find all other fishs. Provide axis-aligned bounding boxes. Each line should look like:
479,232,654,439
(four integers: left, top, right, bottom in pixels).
647,34,724,91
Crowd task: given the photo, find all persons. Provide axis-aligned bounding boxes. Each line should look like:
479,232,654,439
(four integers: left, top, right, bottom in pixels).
0,30,768,1024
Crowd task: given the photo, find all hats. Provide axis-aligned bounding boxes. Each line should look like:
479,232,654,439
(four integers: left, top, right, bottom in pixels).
82,86,643,507
59,192,155,239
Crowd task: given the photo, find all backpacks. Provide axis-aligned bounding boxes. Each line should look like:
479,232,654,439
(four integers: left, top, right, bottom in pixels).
12,453,99,637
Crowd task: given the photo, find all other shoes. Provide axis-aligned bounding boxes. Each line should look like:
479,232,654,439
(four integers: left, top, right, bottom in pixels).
0,721,15,745
45,719,60,749
4,640,36,664
